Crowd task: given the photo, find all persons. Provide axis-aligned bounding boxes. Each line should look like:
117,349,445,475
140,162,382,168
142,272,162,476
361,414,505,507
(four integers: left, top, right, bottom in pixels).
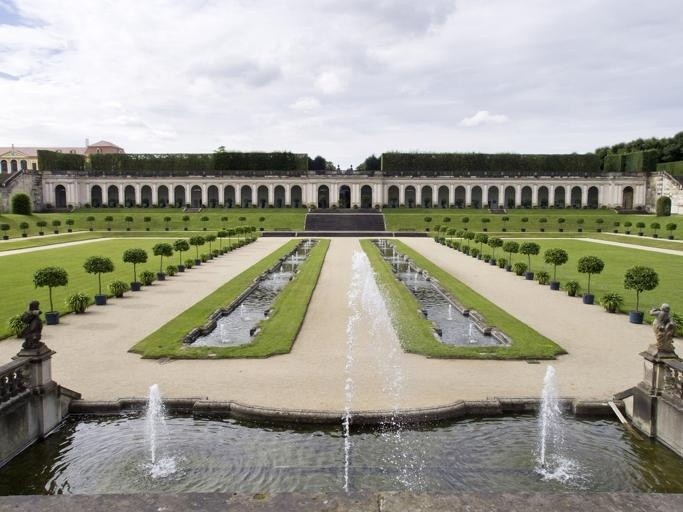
29,300,43,347
650,303,670,353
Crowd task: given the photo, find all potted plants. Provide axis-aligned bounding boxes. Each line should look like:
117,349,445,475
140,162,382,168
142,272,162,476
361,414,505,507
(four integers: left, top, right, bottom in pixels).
424,216,677,325
0,215,267,325
